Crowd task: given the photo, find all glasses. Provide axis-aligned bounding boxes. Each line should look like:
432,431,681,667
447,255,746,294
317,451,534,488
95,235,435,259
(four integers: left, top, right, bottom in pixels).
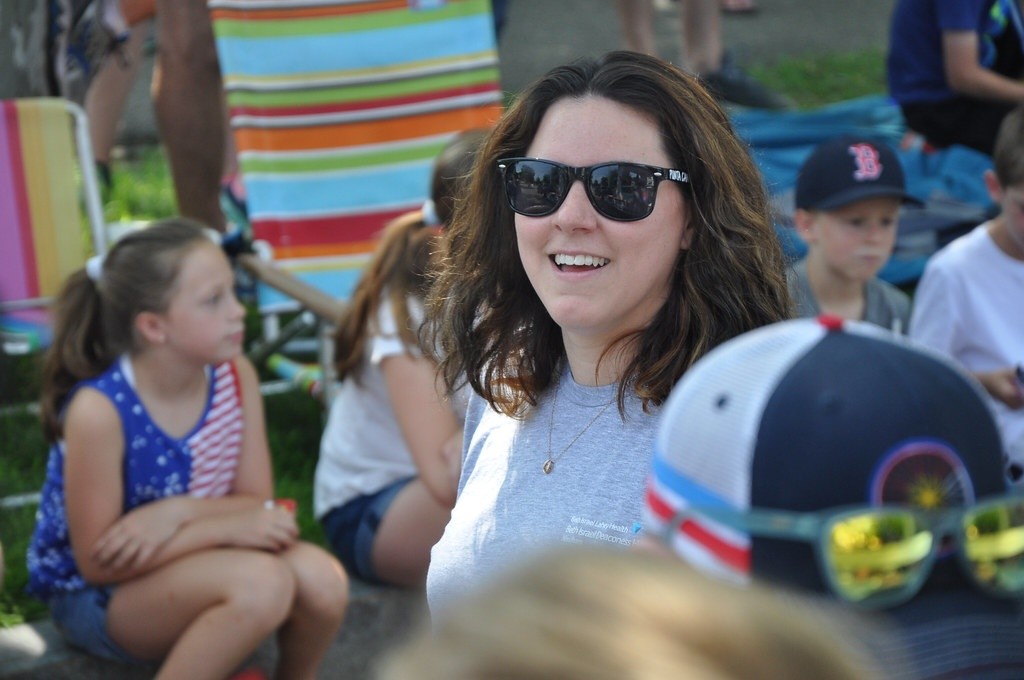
497,157,691,222
816,493,1024,610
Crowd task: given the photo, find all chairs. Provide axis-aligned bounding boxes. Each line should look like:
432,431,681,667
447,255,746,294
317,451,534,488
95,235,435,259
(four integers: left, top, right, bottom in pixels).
0,95,109,514
206,2,509,411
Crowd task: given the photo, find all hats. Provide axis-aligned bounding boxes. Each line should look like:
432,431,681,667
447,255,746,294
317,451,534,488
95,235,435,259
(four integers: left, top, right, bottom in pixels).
645,311,1024,677
797,136,926,214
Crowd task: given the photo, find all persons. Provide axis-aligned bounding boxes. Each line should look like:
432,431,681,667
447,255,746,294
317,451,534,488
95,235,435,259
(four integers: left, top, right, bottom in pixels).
625,0,799,114
84,0,250,255
23,220,349,680
886,0,1024,159
311,127,493,593
425,51,791,624
370,311,1024,680
784,109,1024,490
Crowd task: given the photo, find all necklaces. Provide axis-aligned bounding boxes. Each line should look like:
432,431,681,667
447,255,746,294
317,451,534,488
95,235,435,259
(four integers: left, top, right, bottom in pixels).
542,365,627,474
